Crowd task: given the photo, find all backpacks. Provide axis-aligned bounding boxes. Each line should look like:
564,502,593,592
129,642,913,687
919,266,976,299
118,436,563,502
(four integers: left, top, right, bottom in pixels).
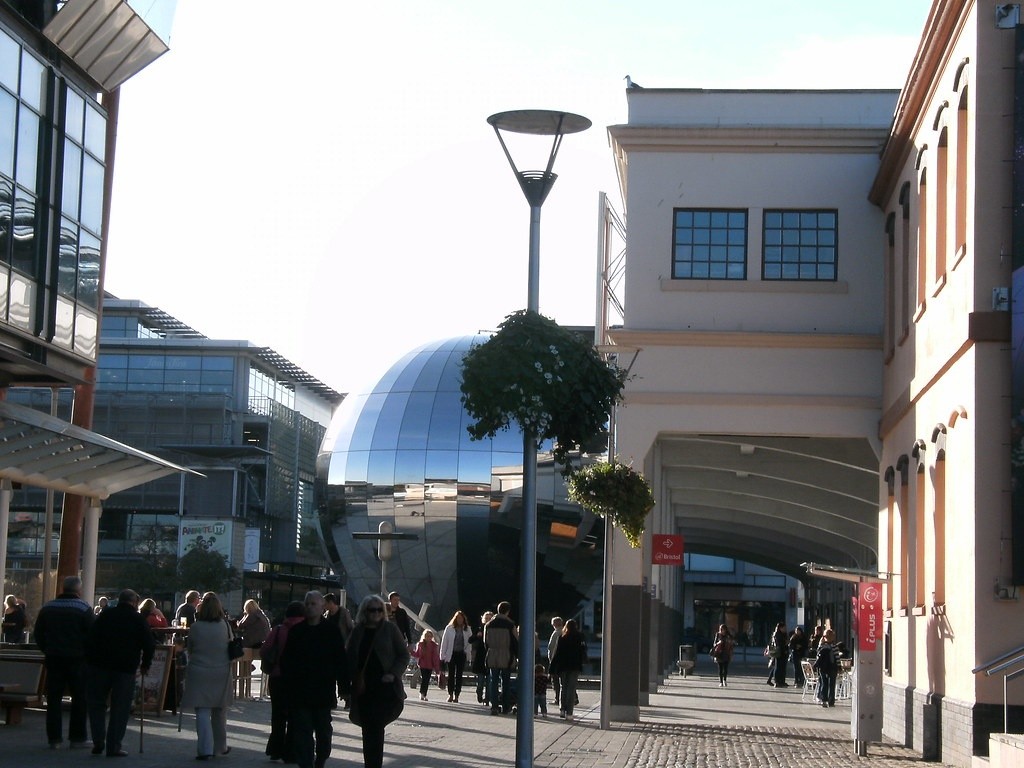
818,645,833,673
764,645,771,656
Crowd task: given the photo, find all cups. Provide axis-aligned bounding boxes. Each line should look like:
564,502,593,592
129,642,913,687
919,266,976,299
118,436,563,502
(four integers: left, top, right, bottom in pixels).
171,619,179,627
180,617,187,629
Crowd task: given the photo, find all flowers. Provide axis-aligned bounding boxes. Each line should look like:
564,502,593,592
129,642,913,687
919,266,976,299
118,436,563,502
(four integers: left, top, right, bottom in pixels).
562,452,656,549
455,306,638,466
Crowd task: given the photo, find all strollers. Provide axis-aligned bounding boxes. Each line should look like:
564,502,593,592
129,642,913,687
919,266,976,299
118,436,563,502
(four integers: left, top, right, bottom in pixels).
496,666,518,714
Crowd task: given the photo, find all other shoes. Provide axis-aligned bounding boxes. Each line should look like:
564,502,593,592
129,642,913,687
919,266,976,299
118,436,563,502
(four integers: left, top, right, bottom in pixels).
220,747,232,754
534,715,543,719
447,694,458,702
766,681,774,686
106,750,128,757
198,755,215,762
719,683,723,687
483,699,489,706
92,747,102,754
70,740,94,749
723,679,727,686
50,743,65,751
490,708,499,716
560,711,565,718
502,706,511,714
543,714,550,720
548,701,558,705
565,714,573,721
476,691,483,703
420,693,428,701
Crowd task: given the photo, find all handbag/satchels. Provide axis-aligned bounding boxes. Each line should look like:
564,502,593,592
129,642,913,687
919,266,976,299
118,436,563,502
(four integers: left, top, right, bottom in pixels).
224,619,245,661
579,632,590,664
348,674,364,699
259,625,280,675
36,663,48,695
769,647,780,657
438,660,446,690
709,632,719,656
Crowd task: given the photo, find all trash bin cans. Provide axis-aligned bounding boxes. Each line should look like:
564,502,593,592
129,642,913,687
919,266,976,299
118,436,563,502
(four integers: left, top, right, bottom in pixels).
679,645,694,676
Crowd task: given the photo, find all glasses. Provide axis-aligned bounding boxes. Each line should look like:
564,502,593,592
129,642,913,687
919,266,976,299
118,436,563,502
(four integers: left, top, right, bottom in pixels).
366,607,384,613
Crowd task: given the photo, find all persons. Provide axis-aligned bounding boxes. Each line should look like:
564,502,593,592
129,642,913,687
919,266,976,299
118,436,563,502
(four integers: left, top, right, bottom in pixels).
87,589,156,757
548,619,587,721
816,629,843,707
260,590,413,768
790,626,808,688
713,624,733,687
411,629,440,700
767,638,775,686
34,575,98,749
233,600,272,663
548,617,579,705
440,611,473,702
468,600,540,715
182,591,235,761
772,622,791,688
0,595,29,643
95,589,201,641
534,665,553,718
808,626,824,689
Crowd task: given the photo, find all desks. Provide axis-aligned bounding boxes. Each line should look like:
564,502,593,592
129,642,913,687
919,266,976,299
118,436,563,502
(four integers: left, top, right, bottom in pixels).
150,626,189,716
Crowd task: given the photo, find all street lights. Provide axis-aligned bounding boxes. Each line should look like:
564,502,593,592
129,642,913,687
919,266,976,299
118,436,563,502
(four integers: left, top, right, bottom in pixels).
486,108,593,768
